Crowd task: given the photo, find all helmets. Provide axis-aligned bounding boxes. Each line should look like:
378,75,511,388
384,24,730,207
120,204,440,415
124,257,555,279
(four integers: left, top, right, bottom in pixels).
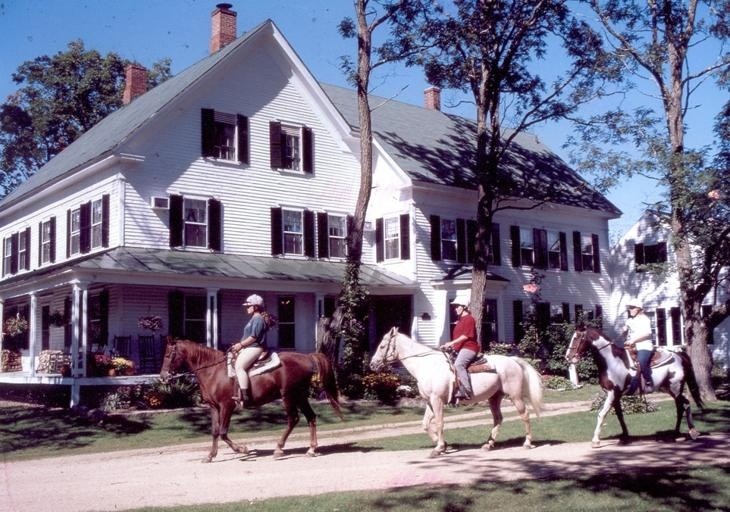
449,298,469,307
242,295,264,306
626,299,644,309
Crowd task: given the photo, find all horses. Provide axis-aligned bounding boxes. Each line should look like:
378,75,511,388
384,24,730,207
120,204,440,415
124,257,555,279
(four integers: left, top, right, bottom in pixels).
369,326,545,455
159,334,345,463
564,320,706,448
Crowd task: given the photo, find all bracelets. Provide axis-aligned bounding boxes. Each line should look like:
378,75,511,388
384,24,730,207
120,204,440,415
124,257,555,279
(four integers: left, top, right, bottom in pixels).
240,342,246,348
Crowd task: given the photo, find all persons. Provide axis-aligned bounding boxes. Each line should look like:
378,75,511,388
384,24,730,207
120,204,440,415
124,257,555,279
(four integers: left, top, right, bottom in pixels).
232,294,267,401
440,296,480,399
618,299,655,393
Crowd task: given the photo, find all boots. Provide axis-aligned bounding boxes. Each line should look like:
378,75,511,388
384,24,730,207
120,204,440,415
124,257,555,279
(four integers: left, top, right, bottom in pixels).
233,389,250,402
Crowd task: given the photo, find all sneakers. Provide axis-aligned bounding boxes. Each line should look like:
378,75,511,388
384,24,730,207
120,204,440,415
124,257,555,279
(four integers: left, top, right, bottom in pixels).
455,391,472,398
645,383,655,394
